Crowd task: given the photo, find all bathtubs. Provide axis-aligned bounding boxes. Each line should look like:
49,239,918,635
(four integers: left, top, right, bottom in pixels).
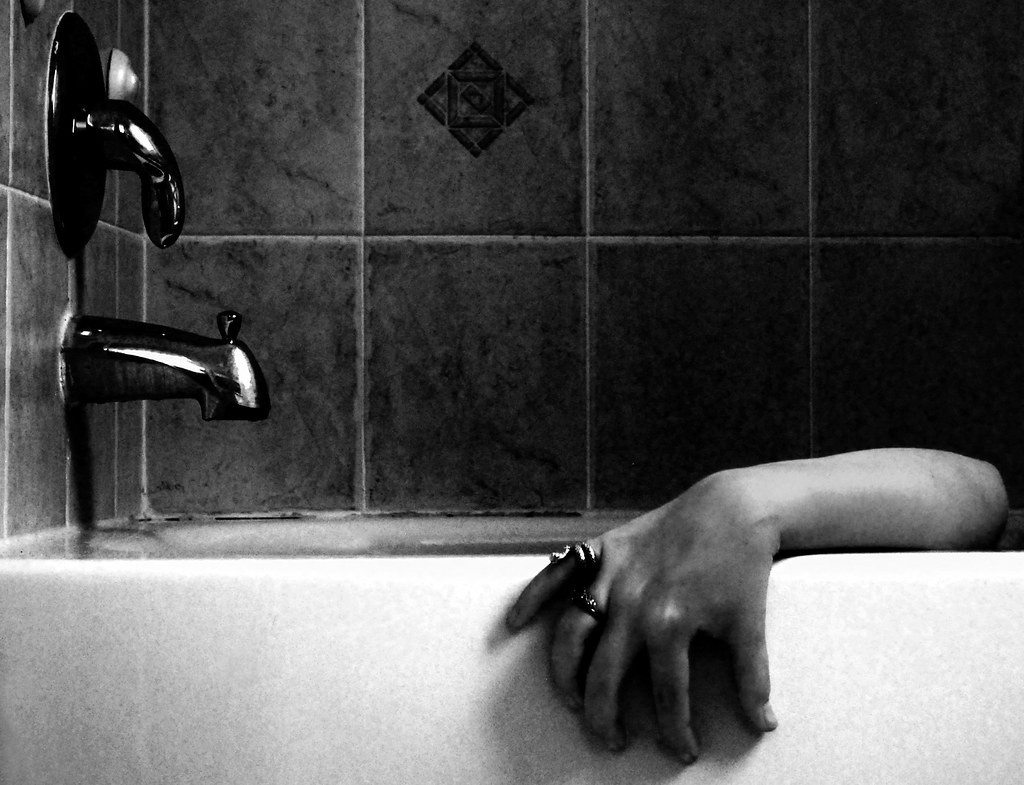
0,510,1024,785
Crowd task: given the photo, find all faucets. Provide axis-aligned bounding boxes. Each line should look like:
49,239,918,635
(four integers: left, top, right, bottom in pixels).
60,302,275,426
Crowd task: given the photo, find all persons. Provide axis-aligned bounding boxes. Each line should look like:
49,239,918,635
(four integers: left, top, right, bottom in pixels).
506,446,1009,764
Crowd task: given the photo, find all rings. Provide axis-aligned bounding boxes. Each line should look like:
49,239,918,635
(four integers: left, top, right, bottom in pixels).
549,540,598,575
559,586,603,621
